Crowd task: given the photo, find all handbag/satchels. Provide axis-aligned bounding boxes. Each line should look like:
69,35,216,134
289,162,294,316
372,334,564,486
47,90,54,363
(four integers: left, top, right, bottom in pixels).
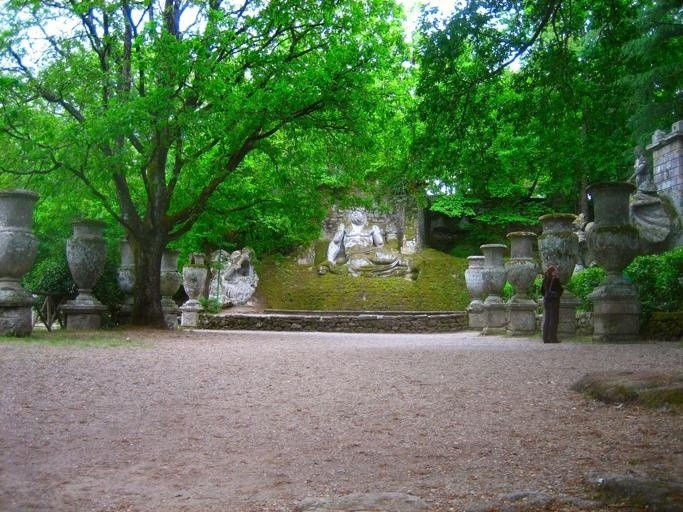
544,289,560,300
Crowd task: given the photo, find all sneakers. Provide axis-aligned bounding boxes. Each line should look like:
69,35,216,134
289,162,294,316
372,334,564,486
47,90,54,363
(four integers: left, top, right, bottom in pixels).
544,338,561,343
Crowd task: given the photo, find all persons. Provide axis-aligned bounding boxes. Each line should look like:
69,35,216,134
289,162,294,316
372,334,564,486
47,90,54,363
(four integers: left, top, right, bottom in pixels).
327,208,415,278
540,266,563,343
627,145,648,193
